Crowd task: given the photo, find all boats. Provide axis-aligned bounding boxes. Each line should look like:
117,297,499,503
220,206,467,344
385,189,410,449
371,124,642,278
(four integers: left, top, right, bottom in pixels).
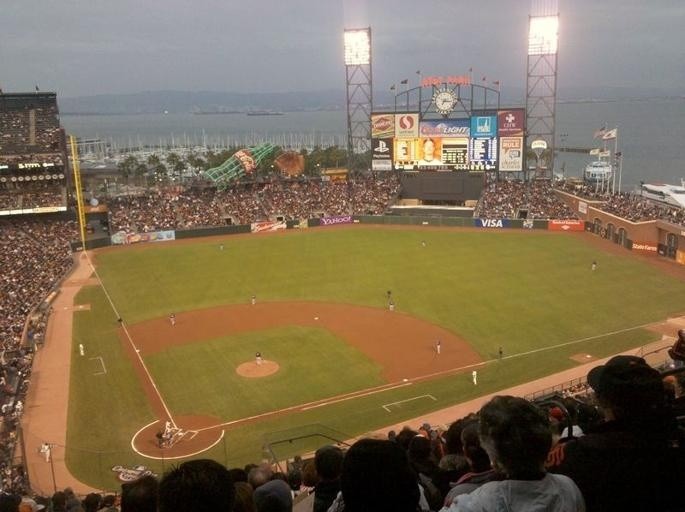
583,160,616,189
631,181,685,210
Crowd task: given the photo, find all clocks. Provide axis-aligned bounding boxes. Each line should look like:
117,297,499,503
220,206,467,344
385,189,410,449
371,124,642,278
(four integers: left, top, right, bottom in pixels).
432,88,457,114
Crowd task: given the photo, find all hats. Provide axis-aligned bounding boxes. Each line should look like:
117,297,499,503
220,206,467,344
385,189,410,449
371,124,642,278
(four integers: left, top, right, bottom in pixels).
586,355,663,407
0,486,46,512
547,405,565,418
303,445,347,487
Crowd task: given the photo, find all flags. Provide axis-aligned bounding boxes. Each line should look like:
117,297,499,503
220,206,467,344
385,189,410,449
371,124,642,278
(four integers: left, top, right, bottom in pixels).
599,150,610,157
594,125,607,138
602,128,617,140
614,152,621,159
590,148,600,155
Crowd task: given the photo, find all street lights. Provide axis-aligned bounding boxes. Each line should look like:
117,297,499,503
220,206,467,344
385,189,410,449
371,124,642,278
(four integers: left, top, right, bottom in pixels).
558,130,569,180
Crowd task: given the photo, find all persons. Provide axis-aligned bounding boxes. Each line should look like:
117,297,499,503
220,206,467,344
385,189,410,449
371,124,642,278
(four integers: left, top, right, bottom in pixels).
0,175,685,511
416,139,441,165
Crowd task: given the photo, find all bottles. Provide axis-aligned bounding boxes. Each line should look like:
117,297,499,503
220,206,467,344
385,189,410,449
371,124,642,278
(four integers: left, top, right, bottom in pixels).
203,141,274,195
